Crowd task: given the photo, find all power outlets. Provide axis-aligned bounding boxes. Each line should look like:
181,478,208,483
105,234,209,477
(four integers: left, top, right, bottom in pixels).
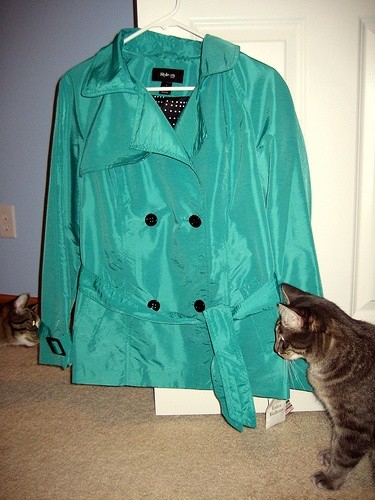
0,203,17,242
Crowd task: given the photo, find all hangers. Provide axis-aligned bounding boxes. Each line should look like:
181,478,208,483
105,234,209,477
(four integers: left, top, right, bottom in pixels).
119,1,205,94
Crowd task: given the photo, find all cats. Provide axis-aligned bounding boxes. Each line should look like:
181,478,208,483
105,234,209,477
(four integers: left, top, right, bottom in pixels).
274,283,375,491
0,293,41,347
